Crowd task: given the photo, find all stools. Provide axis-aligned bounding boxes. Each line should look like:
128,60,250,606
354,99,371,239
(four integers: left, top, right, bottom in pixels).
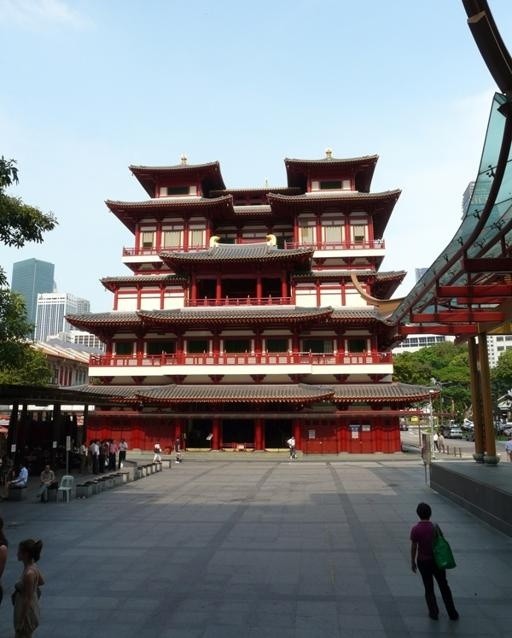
8,486,28,501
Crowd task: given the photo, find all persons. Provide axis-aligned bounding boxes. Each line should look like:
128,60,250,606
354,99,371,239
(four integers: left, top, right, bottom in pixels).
151,438,162,464
10,537,45,638
432,430,439,450
286,434,297,460
410,499,462,620
0,436,129,476
506,434,512,462
173,435,181,463
438,430,446,452
0,514,8,608
0,460,29,501
35,464,55,505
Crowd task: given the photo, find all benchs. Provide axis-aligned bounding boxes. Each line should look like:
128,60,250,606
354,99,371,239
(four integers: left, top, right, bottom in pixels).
126,458,174,468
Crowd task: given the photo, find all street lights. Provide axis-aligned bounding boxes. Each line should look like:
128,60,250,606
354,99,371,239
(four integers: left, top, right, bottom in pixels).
428,389,434,459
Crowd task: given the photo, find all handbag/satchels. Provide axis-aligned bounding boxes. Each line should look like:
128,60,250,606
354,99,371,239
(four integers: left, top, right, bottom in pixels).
431,524,458,572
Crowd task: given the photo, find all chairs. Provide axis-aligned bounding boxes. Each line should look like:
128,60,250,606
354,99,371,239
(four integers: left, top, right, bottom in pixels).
56,475,74,503
40,482,58,502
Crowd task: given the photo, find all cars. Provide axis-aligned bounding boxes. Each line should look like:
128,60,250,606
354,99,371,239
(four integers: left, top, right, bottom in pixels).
410,416,418,422
442,419,463,439
505,432,512,461
